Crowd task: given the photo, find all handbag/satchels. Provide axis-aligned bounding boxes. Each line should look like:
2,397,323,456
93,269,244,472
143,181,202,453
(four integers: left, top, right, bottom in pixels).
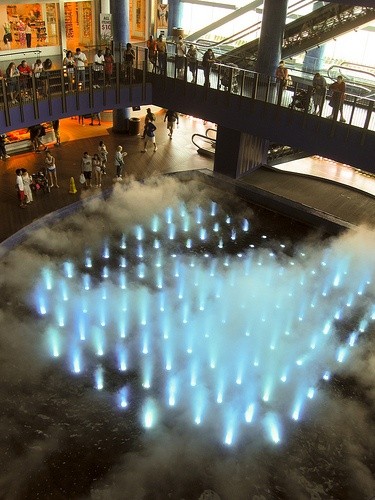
119,160,125,166
79,173,86,184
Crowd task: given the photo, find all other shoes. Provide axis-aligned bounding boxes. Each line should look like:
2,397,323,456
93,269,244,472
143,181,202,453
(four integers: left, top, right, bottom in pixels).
35,150,41,153
20,205,26,209
12,83,112,104
50,185,54,188
101,170,103,174
141,150,146,152
55,142,61,145
98,124,101,126
170,137,172,139
168,133,171,136
89,123,94,125
99,184,101,187
116,175,122,177
5,156,10,158
28,202,31,205
104,172,106,174
96,185,99,187
141,135,145,139
44,146,48,151
55,184,59,188
154,147,157,152
117,177,122,181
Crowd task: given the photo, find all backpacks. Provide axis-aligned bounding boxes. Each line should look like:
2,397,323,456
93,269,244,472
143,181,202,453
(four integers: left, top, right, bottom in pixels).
41,125,46,136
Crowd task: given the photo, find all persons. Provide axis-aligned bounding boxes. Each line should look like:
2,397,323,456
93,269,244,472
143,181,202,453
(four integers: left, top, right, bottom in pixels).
18,21,51,48
0,35,346,123
0,108,217,209
3,22,11,43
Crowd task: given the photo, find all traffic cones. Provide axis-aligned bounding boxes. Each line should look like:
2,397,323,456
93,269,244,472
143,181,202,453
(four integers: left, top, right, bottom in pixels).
68,177,77,194
